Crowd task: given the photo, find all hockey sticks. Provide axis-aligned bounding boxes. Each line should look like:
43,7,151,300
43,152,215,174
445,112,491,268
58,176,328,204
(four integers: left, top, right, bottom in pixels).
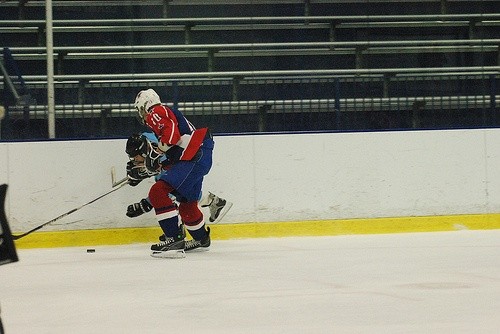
0,180,132,240
111,140,183,188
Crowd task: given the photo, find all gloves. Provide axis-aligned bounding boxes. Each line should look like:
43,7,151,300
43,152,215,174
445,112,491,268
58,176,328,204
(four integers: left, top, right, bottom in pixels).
125,160,148,188
146,146,164,172
126,199,152,218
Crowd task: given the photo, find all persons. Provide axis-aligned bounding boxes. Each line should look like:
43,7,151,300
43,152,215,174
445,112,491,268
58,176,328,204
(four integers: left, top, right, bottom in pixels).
0,92,18,265
133,89,215,258
125,132,234,242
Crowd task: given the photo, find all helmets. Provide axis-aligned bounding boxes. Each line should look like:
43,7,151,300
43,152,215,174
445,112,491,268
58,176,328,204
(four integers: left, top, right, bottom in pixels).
133,89,162,117
125,133,150,162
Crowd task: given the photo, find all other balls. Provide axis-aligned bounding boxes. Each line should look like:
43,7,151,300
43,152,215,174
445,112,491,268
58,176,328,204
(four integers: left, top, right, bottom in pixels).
87,249,95,253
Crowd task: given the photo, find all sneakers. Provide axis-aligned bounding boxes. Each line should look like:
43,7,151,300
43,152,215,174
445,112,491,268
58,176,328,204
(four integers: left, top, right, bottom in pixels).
181,226,214,253
201,191,234,225
158,220,187,242
150,235,186,259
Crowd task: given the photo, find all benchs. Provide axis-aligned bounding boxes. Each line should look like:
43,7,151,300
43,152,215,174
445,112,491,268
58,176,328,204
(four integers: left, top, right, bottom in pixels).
0,14,500,120
